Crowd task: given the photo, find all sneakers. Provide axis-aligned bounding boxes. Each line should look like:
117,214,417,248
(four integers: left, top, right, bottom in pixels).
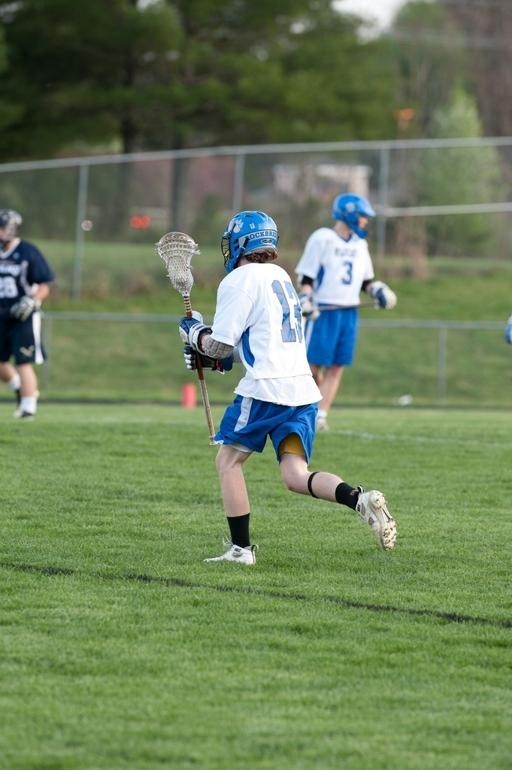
314,416,331,435
353,483,400,552
202,543,259,566
11,384,41,420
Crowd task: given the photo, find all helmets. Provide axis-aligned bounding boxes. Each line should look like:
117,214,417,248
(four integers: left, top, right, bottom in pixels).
0,209,23,240
331,192,377,239
220,210,281,274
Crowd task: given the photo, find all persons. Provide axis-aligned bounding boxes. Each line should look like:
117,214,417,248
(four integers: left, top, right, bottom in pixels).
294,192,397,431
0,207,54,419
178,211,397,564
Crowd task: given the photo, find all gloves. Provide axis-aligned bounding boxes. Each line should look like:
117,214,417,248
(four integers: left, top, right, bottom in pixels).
297,291,321,320
176,309,213,357
365,279,399,312
10,293,42,322
182,344,231,375
